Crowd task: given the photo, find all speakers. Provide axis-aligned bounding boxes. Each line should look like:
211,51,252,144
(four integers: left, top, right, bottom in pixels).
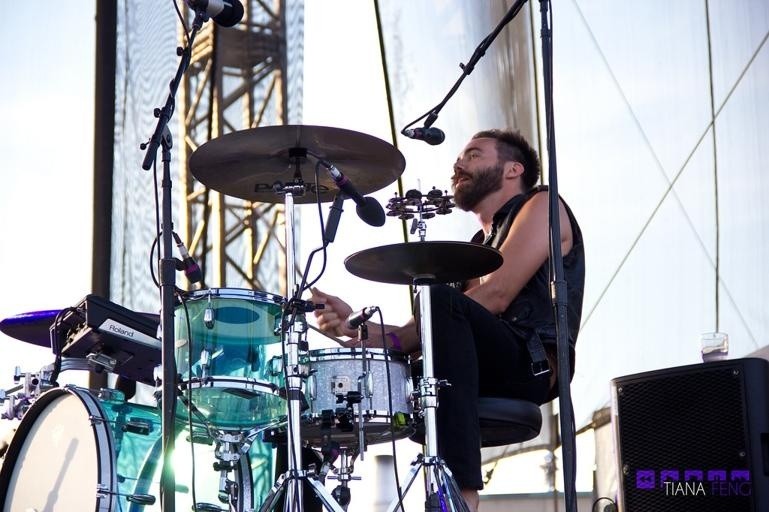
610,357,769,512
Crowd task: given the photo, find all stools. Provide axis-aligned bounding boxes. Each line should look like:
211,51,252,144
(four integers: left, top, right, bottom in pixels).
410,397,542,448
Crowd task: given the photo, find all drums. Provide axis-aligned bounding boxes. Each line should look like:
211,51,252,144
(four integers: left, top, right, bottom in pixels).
172,285,311,428
308,345,418,447
0,387,257,511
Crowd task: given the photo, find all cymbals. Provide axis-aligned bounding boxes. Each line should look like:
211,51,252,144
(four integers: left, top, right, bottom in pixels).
344,240,504,286
189,125,406,205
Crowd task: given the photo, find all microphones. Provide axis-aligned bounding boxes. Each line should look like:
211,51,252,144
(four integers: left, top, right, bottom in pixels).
345,306,379,330
186,1,244,28
402,128,445,145
322,158,385,227
173,232,202,283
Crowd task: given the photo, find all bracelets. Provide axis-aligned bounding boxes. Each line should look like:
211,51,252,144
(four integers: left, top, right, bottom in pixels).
384,327,405,353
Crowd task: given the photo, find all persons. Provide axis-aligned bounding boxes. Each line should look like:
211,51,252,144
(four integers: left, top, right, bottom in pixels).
312,128,585,512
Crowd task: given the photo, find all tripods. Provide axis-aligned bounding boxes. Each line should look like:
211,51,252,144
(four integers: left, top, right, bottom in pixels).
387,221,470,512
258,193,344,512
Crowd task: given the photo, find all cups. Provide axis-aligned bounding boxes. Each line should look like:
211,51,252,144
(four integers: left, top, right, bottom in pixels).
698,332,729,361
